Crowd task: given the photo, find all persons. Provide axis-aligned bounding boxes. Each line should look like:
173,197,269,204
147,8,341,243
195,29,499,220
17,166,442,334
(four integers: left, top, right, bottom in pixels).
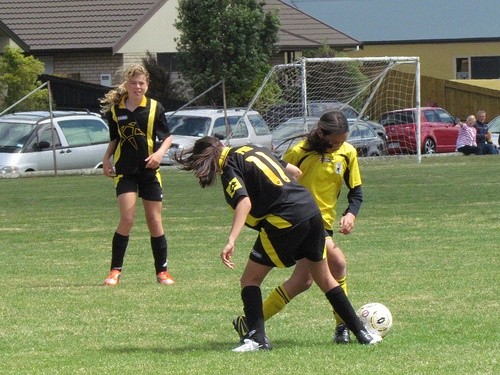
168,137,382,354
455,110,500,155
98,66,176,286
230,112,365,345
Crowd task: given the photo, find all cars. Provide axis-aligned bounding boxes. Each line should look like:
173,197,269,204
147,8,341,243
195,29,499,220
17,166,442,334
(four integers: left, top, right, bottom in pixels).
372,106,460,155
485,115,500,149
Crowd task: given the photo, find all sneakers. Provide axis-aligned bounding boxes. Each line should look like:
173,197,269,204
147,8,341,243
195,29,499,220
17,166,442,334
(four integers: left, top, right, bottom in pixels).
232,316,249,342
334,323,351,344
156,272,174,285
105,269,121,286
356,326,376,345
232,339,271,353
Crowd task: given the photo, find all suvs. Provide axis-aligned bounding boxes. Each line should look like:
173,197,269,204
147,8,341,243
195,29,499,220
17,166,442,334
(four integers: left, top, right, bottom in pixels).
0,111,114,176
155,105,273,165
271,100,390,169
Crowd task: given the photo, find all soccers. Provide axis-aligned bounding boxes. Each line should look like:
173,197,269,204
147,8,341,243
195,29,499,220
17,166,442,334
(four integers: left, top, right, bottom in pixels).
356,302,393,338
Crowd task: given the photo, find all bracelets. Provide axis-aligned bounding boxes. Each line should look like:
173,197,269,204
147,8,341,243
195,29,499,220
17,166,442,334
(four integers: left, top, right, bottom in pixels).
461,124,463,128
